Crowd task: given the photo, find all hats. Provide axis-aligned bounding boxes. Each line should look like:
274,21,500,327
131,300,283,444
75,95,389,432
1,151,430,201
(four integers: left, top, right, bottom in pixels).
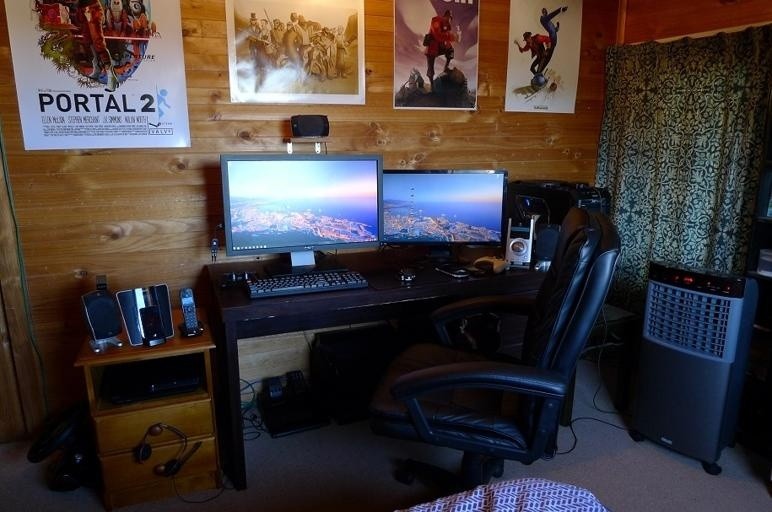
250,12,257,19
523,32,532,41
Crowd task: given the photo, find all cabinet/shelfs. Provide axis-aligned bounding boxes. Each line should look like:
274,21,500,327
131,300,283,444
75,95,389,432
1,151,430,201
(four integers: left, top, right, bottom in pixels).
84,351,223,510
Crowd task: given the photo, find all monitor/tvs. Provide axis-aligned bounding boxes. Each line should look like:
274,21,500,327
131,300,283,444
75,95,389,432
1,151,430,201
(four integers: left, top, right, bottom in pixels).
220,153,383,276
383,169,508,264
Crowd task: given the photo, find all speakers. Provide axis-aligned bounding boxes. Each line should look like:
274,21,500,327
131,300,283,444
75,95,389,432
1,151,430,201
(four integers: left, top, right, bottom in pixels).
80,289,124,353
533,228,559,272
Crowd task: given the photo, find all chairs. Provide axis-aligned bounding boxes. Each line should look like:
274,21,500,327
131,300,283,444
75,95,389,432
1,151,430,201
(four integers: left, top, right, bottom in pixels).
367,207,620,492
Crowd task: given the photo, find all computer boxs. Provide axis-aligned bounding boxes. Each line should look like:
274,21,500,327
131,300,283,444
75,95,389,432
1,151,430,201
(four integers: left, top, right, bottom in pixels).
506,179,612,260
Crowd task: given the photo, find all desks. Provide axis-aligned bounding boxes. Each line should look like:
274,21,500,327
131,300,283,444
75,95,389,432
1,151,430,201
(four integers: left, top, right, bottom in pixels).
205,245,546,487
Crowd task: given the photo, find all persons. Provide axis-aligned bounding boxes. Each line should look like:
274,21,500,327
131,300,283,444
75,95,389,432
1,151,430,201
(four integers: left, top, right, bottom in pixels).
514,31,552,75
69,0,119,92
424,8,461,93
540,5,570,72
245,12,348,81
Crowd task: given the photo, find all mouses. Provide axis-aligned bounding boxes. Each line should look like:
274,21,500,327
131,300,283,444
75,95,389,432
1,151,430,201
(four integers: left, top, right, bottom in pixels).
397,268,417,281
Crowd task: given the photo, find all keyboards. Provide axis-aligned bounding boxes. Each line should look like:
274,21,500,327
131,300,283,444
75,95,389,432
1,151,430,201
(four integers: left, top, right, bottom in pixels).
246,270,368,298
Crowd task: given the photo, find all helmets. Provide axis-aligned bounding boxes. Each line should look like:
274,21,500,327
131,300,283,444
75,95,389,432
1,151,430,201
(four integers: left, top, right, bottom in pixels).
444,9,454,19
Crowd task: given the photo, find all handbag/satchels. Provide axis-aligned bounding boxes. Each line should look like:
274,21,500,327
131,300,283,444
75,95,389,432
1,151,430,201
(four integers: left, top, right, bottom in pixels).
265,44,273,55
424,34,431,46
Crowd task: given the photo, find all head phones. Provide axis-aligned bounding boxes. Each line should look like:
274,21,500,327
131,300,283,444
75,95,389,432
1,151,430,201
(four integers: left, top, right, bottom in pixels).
134,423,189,475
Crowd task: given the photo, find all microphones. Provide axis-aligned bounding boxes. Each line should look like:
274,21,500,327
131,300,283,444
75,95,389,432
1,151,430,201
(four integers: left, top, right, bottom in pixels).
166,441,204,477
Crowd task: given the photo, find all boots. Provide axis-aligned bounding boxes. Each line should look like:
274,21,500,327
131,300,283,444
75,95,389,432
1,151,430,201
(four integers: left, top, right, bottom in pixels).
90,57,105,79
107,67,119,92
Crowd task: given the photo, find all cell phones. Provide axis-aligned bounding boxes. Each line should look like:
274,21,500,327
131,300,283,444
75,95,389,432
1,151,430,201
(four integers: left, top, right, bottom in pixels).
139,304,165,348
